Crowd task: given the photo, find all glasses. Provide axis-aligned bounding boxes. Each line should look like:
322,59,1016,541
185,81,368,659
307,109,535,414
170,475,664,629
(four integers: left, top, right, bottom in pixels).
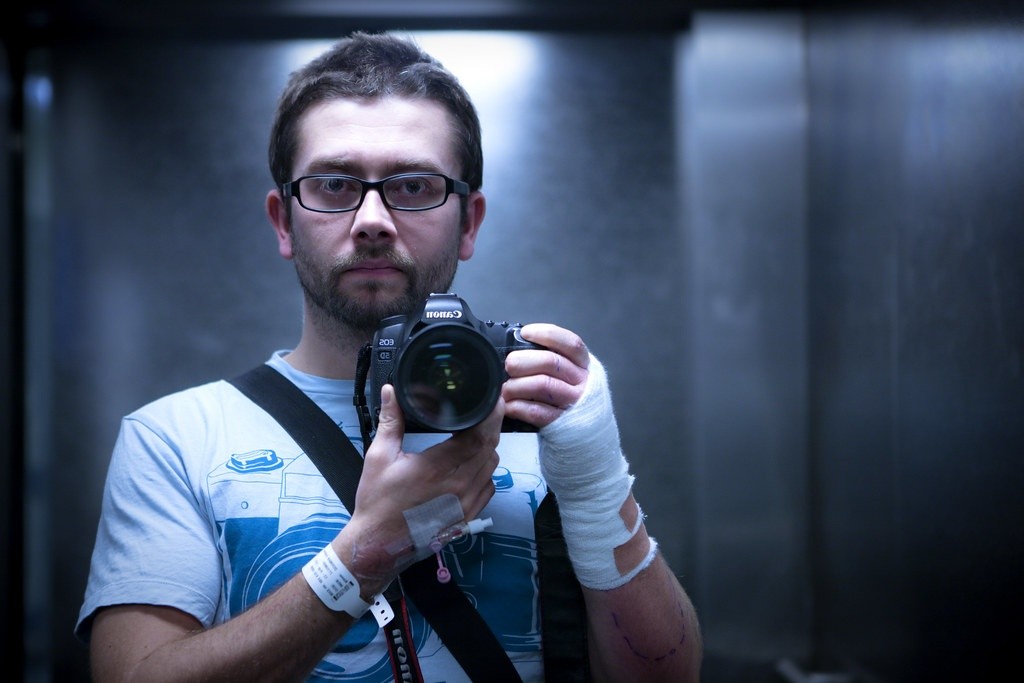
282,172,471,213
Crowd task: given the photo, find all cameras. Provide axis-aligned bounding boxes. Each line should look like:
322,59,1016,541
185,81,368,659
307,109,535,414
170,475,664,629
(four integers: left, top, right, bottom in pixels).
372,291,548,434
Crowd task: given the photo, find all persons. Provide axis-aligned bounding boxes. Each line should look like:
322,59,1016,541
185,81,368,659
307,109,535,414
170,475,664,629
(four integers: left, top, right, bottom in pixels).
72,31,704,683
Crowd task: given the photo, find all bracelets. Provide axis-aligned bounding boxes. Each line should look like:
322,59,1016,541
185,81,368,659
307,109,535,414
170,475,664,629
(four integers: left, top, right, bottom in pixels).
301,542,395,628
